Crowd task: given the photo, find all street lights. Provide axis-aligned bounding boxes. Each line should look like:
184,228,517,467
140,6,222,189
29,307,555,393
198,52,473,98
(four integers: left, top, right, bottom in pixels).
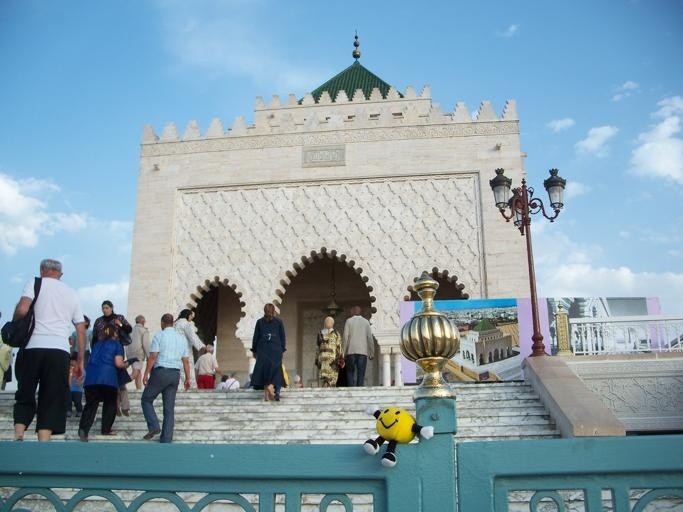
488,167,567,355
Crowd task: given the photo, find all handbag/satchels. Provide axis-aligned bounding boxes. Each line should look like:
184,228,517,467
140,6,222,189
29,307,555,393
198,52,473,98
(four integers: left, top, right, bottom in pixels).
116,314,133,346
0,276,43,349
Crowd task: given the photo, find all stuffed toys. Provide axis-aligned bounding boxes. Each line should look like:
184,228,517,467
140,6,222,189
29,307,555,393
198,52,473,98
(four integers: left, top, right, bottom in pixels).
362,404,434,470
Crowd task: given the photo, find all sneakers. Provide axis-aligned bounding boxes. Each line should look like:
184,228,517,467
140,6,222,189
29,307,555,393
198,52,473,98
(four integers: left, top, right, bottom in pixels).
143,426,161,440
102,430,117,435
78,427,89,443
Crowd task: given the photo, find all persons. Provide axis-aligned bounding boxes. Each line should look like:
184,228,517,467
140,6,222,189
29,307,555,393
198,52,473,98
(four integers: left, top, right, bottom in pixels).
226,371,241,391
313,316,344,387
78,328,138,443
66,351,85,416
140,313,191,418
12,258,85,441
125,315,150,390
216,375,228,389
343,306,375,386
175,308,207,390
67,315,93,361
91,300,132,343
250,304,287,401
195,344,224,389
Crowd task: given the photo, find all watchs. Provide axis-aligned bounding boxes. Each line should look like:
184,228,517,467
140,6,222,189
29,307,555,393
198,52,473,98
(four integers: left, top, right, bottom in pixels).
119,324,123,327
